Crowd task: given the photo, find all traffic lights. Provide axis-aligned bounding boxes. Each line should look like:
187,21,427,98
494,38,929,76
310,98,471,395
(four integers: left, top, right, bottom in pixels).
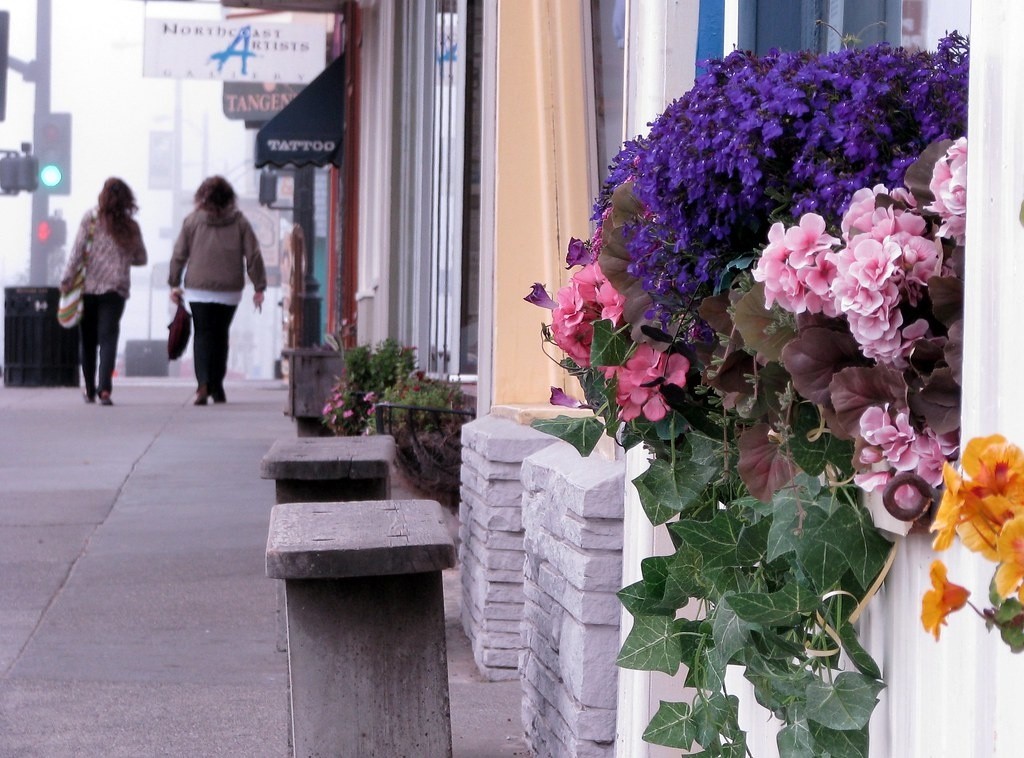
36,112,74,195
34,216,67,248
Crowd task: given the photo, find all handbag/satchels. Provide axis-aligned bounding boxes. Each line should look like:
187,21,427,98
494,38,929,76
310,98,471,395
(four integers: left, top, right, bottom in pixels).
57,267,83,329
168,300,192,361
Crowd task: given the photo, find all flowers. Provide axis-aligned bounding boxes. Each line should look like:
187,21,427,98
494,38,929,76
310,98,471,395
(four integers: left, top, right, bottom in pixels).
324,316,467,435
529,35,1024,758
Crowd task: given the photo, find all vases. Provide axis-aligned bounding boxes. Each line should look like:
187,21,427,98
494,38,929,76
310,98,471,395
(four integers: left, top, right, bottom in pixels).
370,403,475,504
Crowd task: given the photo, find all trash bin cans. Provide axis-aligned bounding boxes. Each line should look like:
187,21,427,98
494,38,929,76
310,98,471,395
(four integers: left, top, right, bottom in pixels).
3,284,83,387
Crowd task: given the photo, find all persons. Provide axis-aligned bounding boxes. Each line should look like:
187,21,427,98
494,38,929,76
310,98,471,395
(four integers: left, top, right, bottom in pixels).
61,177,147,405
168,176,267,406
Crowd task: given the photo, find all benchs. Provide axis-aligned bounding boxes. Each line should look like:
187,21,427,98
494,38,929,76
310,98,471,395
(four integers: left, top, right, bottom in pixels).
259,436,394,503
267,500,456,757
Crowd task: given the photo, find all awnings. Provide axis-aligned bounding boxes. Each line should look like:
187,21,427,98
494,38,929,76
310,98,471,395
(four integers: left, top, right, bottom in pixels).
254,51,346,169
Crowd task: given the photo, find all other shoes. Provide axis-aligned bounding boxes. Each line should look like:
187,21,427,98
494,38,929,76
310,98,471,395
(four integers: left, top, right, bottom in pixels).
82,389,94,404
210,385,226,403
195,383,208,405
101,392,113,405
